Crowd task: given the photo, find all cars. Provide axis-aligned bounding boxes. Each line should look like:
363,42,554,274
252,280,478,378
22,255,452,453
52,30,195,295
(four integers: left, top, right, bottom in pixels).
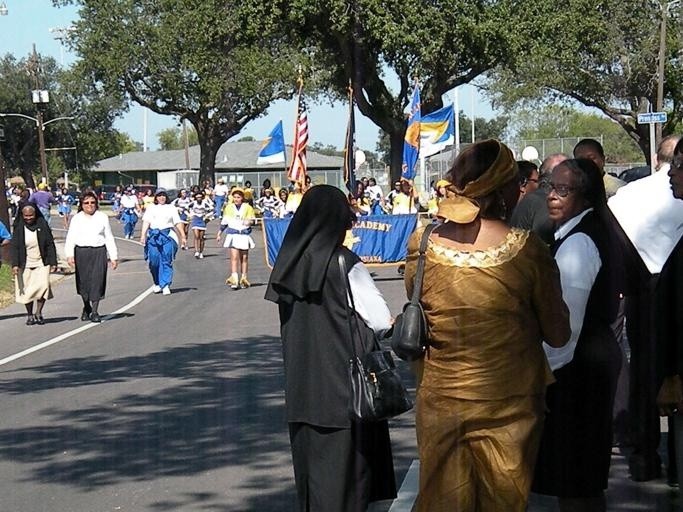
52,183,154,205
617,166,650,183
165,189,190,203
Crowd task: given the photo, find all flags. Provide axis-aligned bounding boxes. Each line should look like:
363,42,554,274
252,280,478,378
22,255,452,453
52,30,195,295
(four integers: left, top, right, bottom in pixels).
256,120,286,165
343,88,356,195
399,83,421,181
418,103,455,159
287,78,308,193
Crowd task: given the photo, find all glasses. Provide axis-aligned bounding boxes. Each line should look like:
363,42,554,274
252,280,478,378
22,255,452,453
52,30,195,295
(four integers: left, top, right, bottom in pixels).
544,183,577,196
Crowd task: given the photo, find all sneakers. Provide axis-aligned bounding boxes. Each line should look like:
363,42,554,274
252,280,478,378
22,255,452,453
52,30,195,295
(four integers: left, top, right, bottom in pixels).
154,285,161,293
231,277,248,289
27,313,45,325
163,286,171,295
89,312,101,322
195,252,204,259
82,306,91,322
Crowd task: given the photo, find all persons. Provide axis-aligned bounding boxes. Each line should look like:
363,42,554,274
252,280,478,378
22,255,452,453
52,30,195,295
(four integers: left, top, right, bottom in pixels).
142,179,258,295
2,183,81,247
263,183,398,511
259,177,450,216
11,202,57,325
114,184,141,239
404,139,682,511
66,193,117,323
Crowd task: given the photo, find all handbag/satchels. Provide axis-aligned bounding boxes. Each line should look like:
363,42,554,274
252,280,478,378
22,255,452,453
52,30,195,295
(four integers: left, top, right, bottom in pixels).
349,351,414,424
391,302,429,361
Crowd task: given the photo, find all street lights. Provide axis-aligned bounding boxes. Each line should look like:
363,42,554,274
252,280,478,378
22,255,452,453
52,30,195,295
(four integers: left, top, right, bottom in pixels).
48,27,77,71
0,112,74,188
